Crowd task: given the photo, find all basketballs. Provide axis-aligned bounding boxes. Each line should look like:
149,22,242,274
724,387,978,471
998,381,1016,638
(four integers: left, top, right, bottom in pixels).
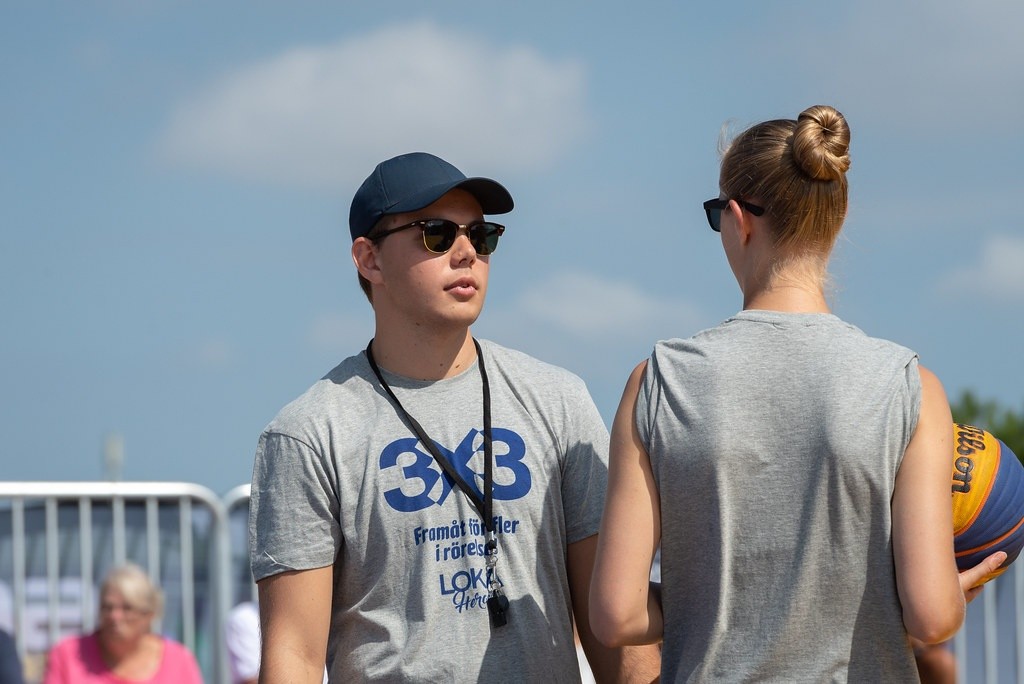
951,423,1024,590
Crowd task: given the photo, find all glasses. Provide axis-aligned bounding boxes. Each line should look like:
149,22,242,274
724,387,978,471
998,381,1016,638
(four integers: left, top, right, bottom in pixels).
369,218,505,256
704,198,765,232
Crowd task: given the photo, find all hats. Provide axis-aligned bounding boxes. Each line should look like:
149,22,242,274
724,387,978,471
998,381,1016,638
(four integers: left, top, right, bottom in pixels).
349,152,514,242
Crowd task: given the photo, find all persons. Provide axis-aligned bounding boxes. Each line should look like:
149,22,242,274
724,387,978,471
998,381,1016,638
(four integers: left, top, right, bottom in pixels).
43,567,204,684
1,582,24,684
589,105,1008,684
248,152,661,684
225,601,328,684
910,635,957,684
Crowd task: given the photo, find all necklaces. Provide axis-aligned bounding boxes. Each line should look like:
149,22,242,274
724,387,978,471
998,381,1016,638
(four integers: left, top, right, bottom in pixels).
367,337,509,628
